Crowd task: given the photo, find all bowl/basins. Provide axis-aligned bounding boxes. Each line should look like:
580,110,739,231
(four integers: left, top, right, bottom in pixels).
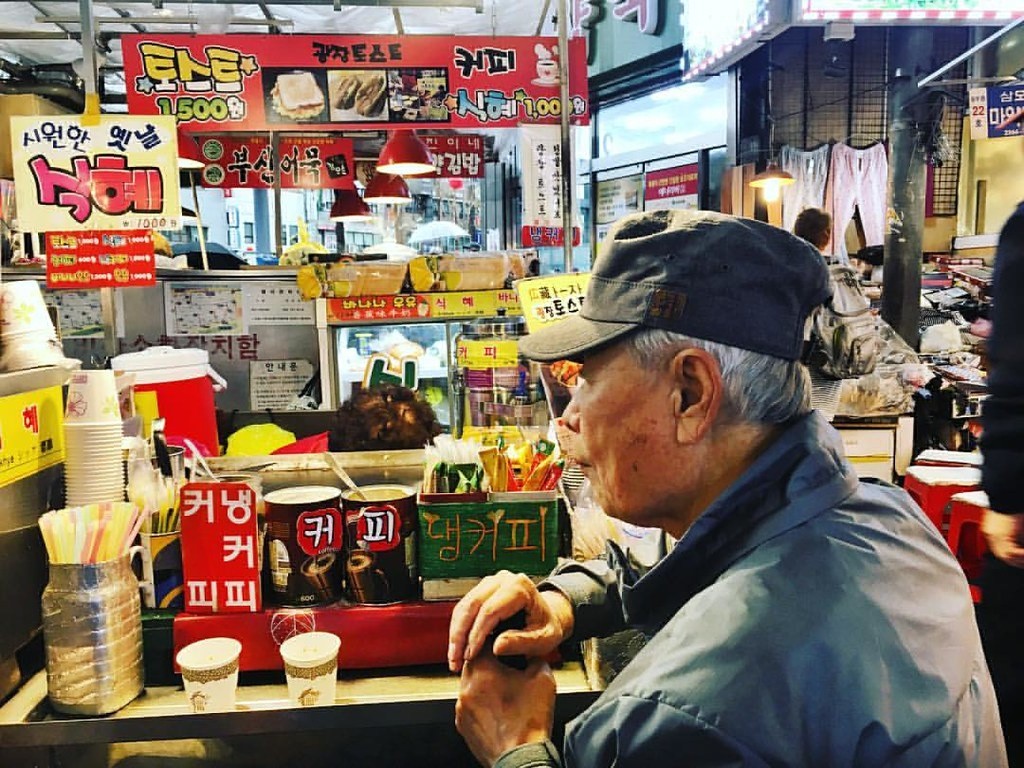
435,251,514,291
326,260,412,296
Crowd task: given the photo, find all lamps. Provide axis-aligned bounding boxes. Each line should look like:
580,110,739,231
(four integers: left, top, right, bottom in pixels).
329,184,375,222
750,163,796,201
376,129,437,174
362,173,412,203
71,50,106,78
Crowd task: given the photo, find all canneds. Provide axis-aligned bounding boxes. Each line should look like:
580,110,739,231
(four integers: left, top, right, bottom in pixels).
263,485,344,608
341,484,421,606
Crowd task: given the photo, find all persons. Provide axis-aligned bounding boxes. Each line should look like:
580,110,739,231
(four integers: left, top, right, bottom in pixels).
447,209,1014,768
983,200,1024,768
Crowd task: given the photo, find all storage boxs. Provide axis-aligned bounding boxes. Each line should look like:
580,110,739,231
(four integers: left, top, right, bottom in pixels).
417,480,558,602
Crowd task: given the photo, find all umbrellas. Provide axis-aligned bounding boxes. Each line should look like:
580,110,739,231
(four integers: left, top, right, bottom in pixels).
163,235,251,270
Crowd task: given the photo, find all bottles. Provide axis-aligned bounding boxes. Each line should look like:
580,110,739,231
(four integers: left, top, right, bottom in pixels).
448,308,550,445
41,552,146,719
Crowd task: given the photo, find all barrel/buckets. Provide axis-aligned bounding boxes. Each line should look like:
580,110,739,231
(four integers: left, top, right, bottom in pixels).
109,343,228,461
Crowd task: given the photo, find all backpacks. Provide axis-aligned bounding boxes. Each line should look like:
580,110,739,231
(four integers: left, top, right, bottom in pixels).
810,258,876,375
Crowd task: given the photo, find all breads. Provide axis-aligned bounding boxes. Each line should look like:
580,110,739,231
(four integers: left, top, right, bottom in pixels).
277,73,322,109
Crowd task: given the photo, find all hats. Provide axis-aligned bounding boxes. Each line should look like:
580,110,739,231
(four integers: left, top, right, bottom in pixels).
517,205,828,362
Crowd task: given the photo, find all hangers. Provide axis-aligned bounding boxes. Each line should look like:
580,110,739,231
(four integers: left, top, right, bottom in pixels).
788,133,825,147
840,133,880,144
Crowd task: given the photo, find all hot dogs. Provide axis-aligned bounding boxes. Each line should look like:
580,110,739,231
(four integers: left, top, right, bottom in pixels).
333,74,386,117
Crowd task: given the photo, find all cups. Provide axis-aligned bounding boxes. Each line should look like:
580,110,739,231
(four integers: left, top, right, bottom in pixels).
264,484,342,608
340,484,420,607
0,280,61,372
279,632,343,708
176,637,243,714
121,444,185,501
138,530,184,608
61,370,128,508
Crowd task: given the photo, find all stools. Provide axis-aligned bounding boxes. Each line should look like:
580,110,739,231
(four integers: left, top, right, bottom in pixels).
905,449,989,599
